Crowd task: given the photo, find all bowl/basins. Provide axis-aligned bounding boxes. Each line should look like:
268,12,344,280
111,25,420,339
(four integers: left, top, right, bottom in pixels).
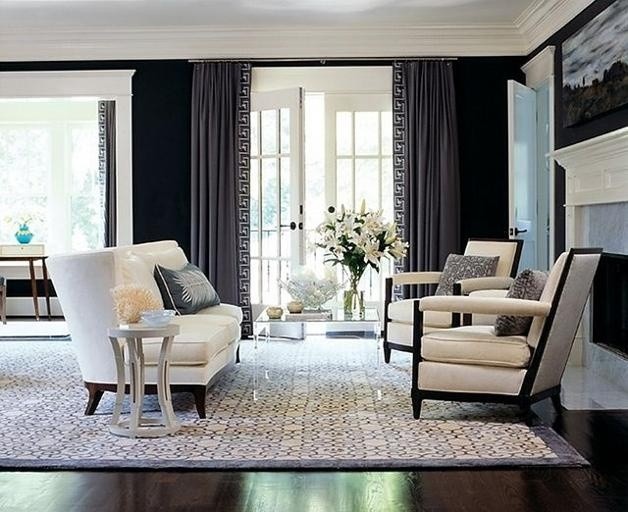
141,309,176,328
266,301,303,319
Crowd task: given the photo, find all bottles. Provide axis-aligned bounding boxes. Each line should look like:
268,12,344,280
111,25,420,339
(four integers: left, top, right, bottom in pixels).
349,284,360,315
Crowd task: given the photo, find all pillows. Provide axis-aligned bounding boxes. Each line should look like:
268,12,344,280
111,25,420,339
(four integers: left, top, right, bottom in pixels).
497,269,546,336
434,253,500,295
155,261,221,314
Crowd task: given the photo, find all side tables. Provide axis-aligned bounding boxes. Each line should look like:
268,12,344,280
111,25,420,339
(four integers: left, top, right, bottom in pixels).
108,325,180,438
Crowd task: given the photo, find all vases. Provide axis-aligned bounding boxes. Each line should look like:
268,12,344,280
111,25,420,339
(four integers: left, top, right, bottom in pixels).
343,290,366,315
16,226,35,244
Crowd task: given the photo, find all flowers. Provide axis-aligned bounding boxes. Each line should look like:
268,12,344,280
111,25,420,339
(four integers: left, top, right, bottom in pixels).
5,213,44,234
112,287,160,322
312,199,408,310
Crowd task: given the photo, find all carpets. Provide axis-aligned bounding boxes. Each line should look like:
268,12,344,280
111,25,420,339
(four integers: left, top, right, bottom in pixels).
2,333,594,470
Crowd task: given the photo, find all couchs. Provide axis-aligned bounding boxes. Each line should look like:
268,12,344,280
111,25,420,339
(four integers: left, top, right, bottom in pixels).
46,240,244,419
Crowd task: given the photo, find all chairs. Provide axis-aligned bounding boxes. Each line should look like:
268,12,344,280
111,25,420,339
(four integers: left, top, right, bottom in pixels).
386,237,525,365
413,248,602,418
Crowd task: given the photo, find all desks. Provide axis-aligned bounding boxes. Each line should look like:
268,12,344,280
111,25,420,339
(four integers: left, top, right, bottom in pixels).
1,245,50,325
